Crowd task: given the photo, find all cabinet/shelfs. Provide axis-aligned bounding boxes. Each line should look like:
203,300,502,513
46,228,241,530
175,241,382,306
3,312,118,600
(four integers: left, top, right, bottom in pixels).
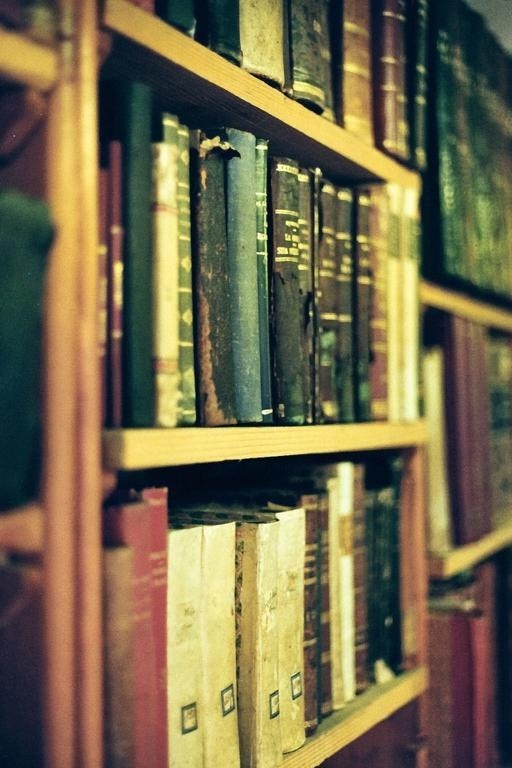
0,0,511,768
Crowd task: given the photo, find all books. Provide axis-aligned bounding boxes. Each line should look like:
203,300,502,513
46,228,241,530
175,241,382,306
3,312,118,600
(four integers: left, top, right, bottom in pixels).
1,0,512,761
